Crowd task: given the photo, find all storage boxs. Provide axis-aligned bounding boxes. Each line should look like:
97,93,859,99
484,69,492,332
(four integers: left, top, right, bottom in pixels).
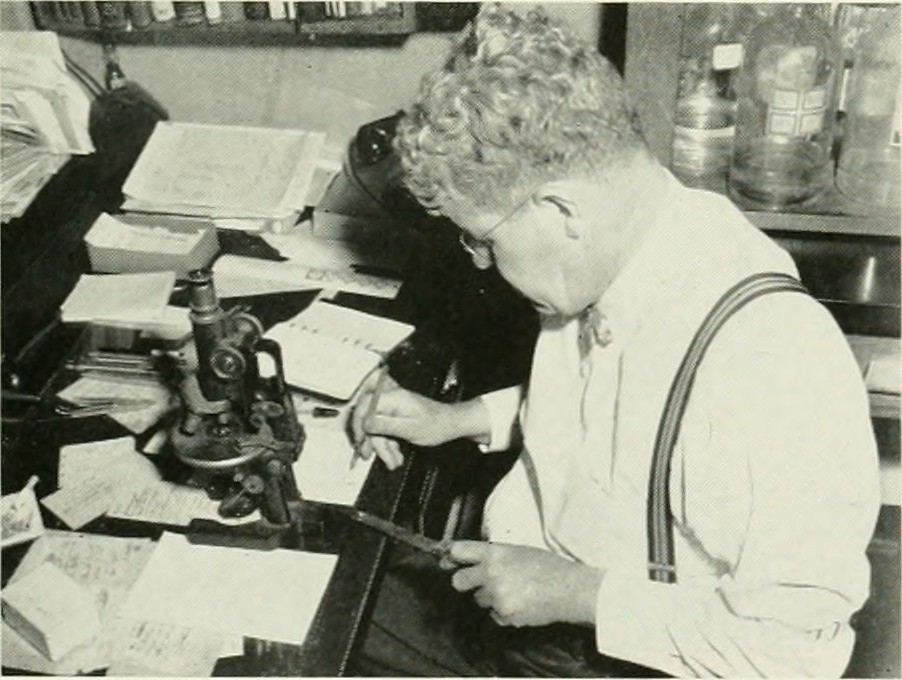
89,216,221,277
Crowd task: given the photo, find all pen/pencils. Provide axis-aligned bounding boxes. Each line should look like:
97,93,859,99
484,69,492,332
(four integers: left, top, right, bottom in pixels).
348,365,388,468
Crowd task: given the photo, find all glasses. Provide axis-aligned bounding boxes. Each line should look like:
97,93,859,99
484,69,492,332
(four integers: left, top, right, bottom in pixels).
459,189,577,263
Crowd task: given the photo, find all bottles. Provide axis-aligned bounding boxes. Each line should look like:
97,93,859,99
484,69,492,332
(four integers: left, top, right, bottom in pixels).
730,2,844,209
672,2,741,192
103,43,128,91
459,232,514,349
836,3,902,207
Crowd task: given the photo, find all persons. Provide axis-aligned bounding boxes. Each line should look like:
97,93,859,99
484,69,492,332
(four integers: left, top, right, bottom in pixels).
345,5,882,677
